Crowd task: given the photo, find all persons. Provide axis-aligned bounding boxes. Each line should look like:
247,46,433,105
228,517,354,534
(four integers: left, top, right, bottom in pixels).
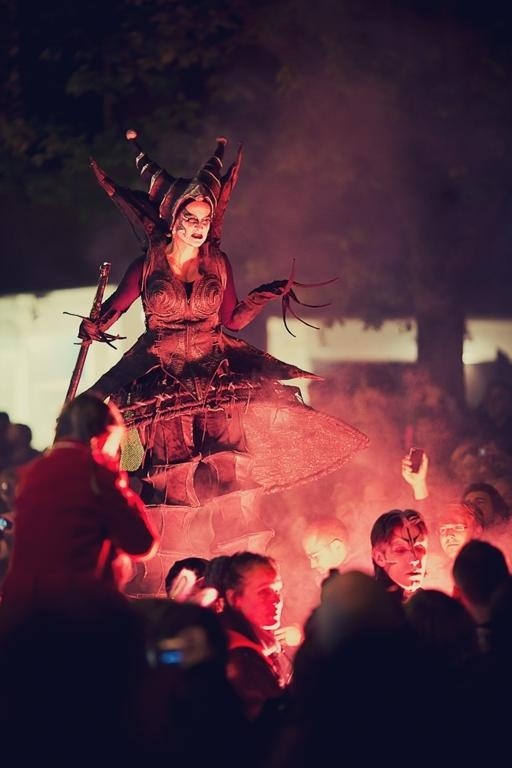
78,200,287,595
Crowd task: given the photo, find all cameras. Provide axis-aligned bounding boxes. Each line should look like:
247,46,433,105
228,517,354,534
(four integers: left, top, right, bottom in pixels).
409,448,424,474
